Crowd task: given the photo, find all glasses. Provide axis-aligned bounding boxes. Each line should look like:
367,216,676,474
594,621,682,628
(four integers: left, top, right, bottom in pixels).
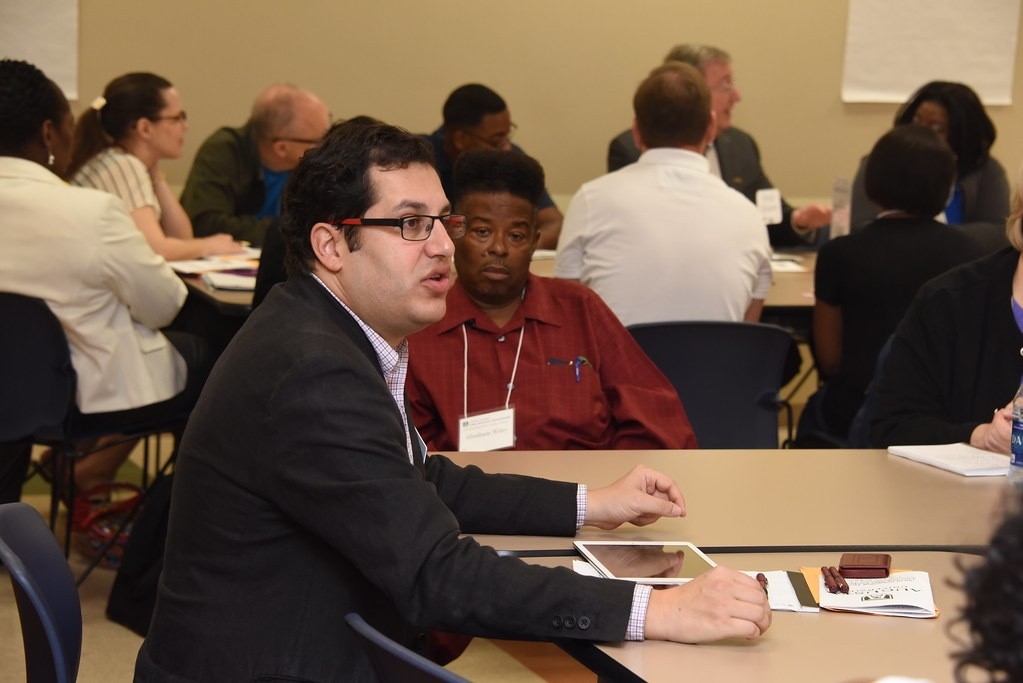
132,111,186,130
459,122,518,146
313,214,468,242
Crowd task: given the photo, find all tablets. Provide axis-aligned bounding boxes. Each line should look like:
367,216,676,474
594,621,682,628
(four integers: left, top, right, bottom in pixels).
572,541,718,585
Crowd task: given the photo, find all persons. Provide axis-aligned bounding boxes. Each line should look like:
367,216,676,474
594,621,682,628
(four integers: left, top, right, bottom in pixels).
421,83,565,250
403,149,695,453
850,82,1010,234
555,60,771,325
584,545,684,578
793,124,980,448
133,116,772,683
179,84,333,248
608,44,833,251
847,166,1023,454
1,57,250,517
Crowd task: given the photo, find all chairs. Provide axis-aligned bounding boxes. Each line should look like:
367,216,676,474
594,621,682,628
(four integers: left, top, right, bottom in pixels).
344,610,478,683
626,320,815,451
0,290,189,683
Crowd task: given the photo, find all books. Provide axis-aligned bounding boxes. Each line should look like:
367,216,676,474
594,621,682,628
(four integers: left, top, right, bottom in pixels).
888,442,1012,476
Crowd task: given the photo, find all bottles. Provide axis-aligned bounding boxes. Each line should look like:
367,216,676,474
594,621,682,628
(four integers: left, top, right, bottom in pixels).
1007,382,1023,485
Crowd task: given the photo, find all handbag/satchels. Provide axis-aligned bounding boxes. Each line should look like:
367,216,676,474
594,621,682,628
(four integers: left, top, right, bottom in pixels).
71,480,142,570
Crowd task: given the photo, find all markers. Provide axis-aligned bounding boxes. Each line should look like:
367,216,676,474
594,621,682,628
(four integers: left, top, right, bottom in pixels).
820,567,838,593
757,573,768,599
828,567,850,596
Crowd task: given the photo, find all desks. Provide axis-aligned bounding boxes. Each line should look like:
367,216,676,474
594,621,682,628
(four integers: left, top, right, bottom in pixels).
425,445,1023,683
179,243,818,384
